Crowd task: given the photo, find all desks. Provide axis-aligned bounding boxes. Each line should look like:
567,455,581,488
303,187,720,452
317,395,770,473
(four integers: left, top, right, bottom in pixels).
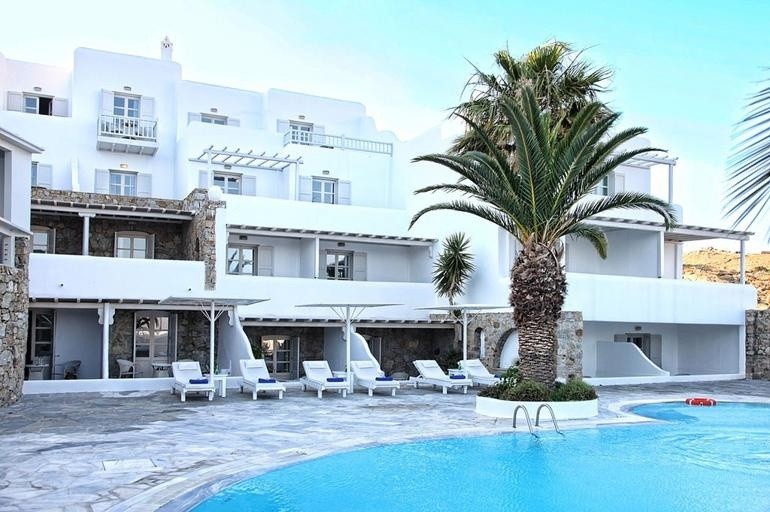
25,364,49,380
204,373,229,398
447,368,469,389
151,362,172,377
332,370,355,394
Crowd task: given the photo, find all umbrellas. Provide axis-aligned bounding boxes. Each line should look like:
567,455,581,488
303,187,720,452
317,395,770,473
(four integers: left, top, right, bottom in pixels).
296,301,404,393
158,290,271,386
413,304,514,393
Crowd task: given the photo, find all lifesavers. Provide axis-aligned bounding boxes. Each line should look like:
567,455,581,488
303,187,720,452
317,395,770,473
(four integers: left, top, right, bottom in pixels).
686,398,716,406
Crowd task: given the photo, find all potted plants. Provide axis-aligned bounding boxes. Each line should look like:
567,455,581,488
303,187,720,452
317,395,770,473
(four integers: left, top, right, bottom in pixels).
472,379,598,421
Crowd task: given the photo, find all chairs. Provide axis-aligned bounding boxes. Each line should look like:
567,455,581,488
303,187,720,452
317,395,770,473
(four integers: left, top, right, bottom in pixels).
117,359,145,378
457,359,505,388
300,361,350,398
238,359,286,400
54,360,82,379
412,360,473,395
169,361,214,402
351,359,401,397
